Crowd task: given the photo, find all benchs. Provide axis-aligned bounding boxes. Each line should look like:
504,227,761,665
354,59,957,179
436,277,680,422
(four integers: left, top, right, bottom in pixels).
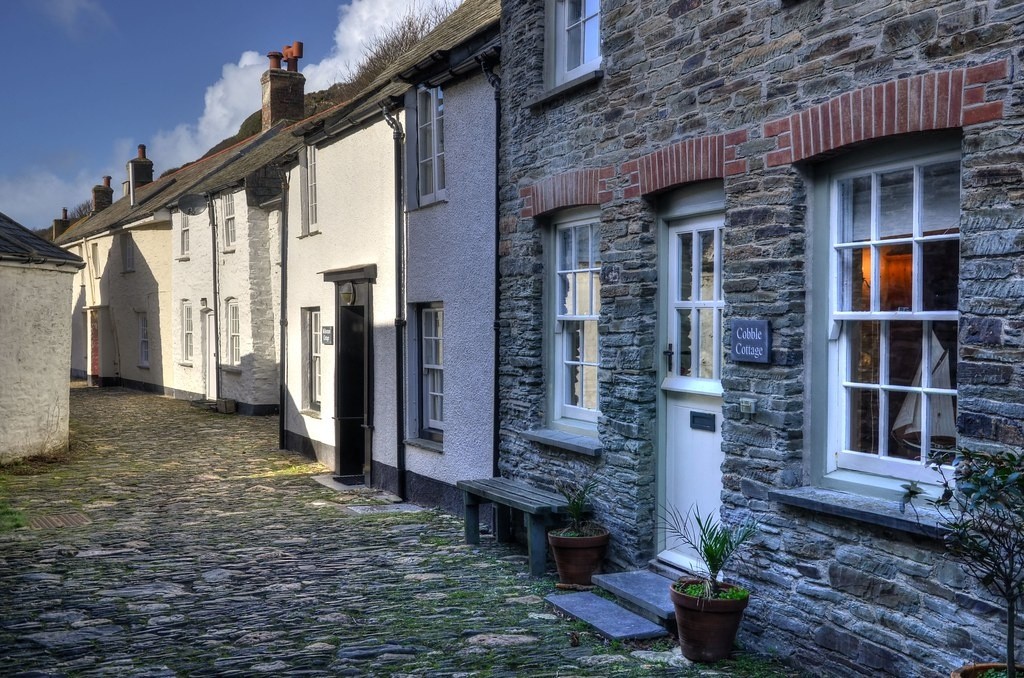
456,476,594,577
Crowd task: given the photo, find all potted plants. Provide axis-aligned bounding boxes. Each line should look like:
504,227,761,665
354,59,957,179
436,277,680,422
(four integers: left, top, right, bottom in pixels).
646,496,774,661
899,444,1024,678
546,475,605,587
